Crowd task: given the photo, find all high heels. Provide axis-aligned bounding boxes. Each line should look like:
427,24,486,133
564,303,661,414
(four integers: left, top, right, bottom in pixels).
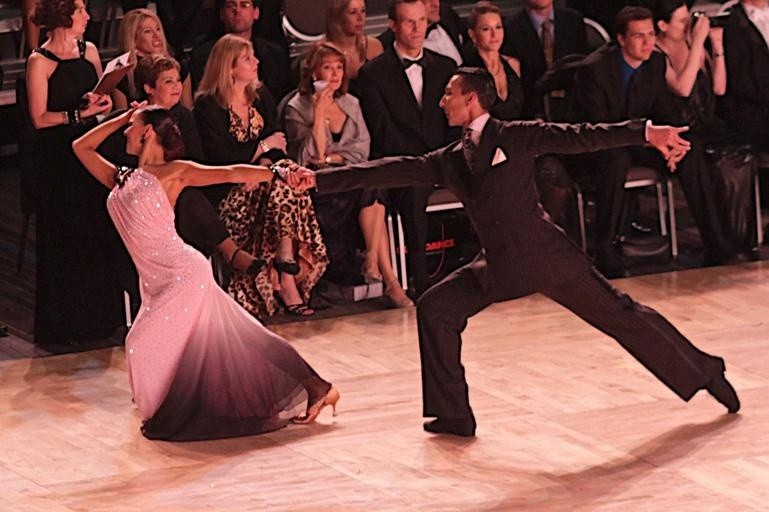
231,248,266,274
274,288,315,318
290,384,340,424
273,257,300,284
385,278,414,308
362,261,383,285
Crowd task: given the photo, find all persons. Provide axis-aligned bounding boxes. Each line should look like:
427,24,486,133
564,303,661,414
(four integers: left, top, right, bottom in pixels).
72,100,340,439
503,0,588,80
286,41,413,307
25,0,112,347
134,54,266,275
296,68,741,437
700,1,769,137
358,0,458,292
193,1,290,99
113,8,167,110
652,0,761,250
312,0,383,79
376,1,468,67
461,1,524,120
193,34,329,326
562,7,759,277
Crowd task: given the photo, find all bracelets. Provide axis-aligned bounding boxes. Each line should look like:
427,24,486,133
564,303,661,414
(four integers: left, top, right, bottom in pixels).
61,110,82,123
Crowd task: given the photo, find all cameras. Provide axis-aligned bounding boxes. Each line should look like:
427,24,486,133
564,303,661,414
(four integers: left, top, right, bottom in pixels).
688,9,732,31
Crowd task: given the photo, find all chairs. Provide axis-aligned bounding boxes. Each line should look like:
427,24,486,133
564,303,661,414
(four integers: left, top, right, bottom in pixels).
545,68,679,261
12,75,37,277
280,90,399,284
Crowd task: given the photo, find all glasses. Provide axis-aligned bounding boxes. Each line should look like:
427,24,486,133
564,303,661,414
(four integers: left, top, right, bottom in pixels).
224,1,252,8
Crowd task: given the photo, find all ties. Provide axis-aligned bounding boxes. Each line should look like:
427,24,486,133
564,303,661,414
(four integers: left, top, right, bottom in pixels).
629,70,639,116
542,19,565,98
463,127,478,173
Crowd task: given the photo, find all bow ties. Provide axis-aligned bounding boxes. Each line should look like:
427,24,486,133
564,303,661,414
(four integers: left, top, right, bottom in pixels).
402,57,425,68
425,22,437,38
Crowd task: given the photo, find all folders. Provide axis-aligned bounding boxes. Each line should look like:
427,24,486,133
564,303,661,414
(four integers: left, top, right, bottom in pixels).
77,48,139,110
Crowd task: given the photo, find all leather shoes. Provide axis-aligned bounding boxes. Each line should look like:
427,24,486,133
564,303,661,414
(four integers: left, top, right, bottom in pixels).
707,247,745,262
423,417,476,436
711,354,740,414
597,252,624,278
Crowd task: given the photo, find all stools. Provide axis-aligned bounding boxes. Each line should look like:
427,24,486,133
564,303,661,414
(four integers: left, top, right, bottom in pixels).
747,151,769,246
396,182,463,292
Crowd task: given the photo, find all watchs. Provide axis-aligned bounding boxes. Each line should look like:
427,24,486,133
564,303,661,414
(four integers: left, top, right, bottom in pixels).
259,140,270,155
323,152,333,165
